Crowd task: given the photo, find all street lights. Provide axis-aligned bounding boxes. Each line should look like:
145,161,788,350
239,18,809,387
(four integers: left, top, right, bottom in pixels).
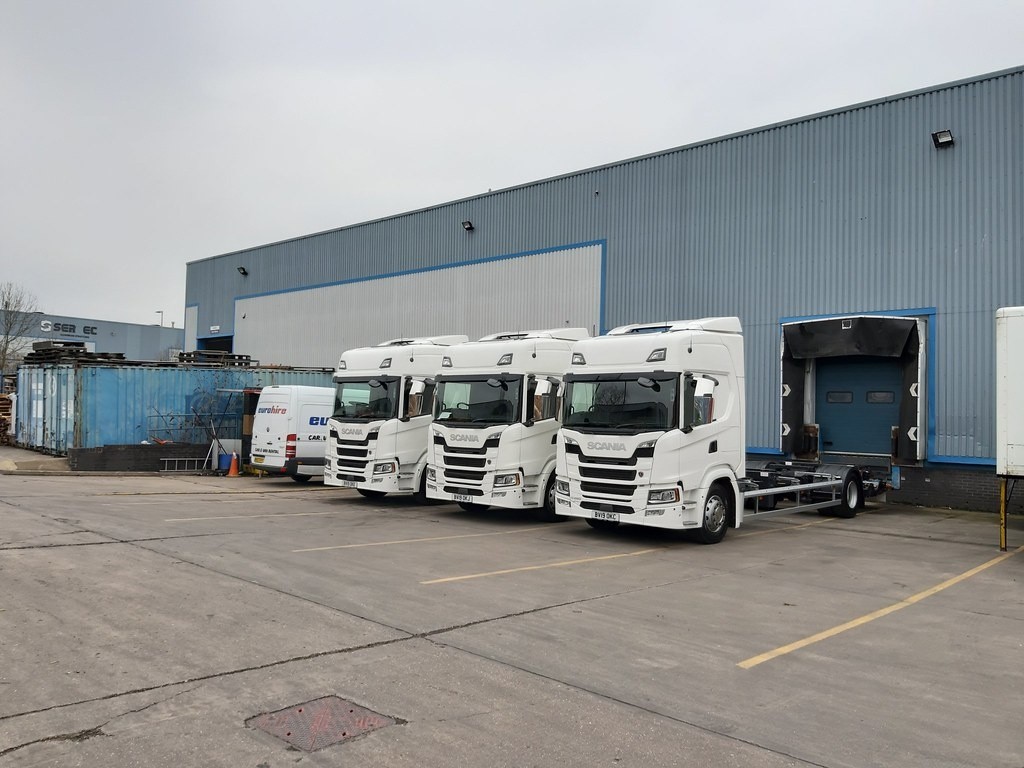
155,310,163,327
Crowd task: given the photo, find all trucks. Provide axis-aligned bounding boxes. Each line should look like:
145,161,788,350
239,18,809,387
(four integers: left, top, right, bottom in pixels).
555,317,896,544
322,334,469,506
426,327,591,522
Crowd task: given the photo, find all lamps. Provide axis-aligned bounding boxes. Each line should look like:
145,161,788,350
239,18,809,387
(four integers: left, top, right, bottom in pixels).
932,128,954,149
237,267,248,275
462,221,474,231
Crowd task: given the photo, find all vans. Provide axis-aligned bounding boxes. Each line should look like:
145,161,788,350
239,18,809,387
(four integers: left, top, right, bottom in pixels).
249,384,370,483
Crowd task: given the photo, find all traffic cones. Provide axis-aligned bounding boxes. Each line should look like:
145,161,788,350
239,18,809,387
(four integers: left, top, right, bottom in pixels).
225,449,242,477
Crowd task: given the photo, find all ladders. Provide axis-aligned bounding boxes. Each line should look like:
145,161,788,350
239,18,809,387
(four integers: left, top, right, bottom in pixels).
158,457,250,472
